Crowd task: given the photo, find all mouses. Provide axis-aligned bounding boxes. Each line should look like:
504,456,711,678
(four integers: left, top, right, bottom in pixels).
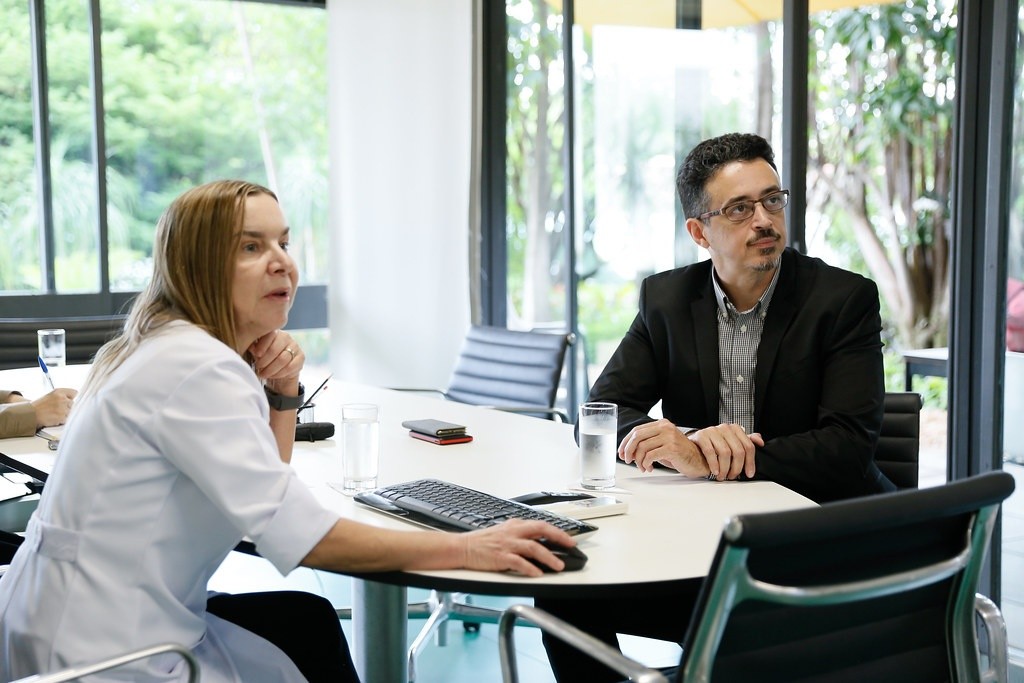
521,538,588,573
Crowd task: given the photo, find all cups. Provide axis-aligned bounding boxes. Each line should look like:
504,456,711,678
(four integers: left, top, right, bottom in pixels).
37,329,66,387
341,403,378,496
578,401,618,491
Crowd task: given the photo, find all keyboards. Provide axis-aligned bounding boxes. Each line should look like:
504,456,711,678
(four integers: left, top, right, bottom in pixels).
354,477,598,547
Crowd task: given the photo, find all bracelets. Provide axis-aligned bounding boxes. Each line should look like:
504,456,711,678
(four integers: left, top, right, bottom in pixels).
263,381,305,411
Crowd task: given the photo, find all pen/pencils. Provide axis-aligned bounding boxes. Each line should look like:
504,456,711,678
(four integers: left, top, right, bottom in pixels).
38,355,56,391
297,372,335,418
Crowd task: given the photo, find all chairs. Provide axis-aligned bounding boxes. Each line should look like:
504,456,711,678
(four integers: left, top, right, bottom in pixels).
499,470,1016,683
330,322,578,683
871,390,922,490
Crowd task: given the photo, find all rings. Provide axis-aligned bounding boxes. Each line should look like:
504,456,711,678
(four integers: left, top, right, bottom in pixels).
285,348,295,359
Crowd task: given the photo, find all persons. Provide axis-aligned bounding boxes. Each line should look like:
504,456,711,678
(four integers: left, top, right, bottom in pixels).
534,132,900,683
0,387,78,438
0,181,578,682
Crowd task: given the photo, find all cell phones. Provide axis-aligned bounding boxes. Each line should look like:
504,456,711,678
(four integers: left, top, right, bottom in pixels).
403,418,474,445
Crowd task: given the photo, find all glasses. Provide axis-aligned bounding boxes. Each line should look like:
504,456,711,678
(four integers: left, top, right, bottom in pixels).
698,189,789,222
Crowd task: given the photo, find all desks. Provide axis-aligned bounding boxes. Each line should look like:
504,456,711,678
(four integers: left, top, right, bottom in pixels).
0,359,825,683
901,344,954,393
